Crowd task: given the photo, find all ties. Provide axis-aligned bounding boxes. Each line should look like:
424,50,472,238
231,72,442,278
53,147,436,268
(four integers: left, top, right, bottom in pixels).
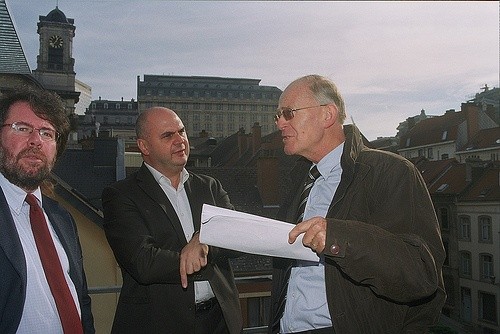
272,164,323,334
25,193,83,334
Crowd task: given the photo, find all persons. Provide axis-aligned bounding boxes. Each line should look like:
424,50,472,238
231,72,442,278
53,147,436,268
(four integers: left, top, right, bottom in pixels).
102,106,244,334
269,75,447,334
0,89,95,334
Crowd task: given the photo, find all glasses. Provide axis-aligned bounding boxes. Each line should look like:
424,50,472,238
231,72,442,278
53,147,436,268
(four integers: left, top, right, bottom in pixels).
272,103,327,125
0,121,59,140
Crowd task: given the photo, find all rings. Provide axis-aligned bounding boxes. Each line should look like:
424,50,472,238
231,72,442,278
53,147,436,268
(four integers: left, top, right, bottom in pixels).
311,240,318,246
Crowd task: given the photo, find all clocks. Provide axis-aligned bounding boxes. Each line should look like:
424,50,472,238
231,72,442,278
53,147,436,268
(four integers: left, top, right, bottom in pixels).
49,35,64,48
